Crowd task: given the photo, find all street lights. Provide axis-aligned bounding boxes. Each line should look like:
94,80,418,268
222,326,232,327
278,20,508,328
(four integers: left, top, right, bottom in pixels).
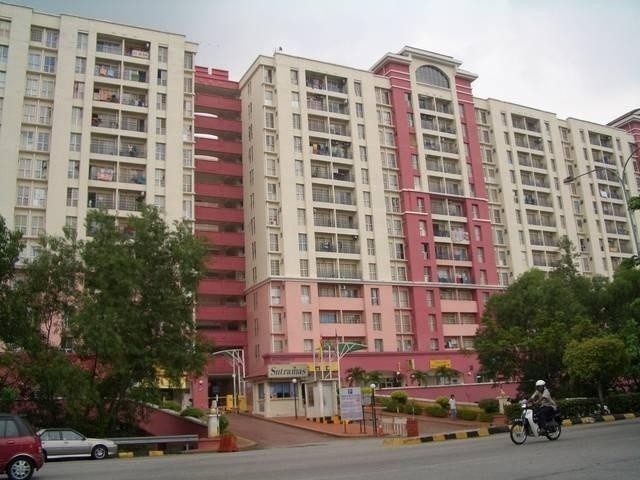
371,384,377,435
563,142,640,271
232,374,238,408
291,379,299,420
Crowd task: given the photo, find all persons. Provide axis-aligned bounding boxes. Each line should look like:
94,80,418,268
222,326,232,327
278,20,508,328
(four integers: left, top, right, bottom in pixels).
523,379,559,436
448,394,459,420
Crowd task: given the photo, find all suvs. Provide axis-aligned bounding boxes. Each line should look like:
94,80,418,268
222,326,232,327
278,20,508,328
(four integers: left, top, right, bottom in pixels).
0,415,47,480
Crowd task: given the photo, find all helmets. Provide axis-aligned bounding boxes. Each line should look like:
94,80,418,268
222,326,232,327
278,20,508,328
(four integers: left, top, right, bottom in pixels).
535,380,547,386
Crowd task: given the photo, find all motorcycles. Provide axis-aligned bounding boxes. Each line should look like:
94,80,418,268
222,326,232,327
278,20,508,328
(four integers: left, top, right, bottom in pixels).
510,399,561,445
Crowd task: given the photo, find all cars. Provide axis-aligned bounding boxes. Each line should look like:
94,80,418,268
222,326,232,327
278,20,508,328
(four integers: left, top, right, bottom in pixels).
563,397,612,418
57,347,76,355
36,428,120,462
